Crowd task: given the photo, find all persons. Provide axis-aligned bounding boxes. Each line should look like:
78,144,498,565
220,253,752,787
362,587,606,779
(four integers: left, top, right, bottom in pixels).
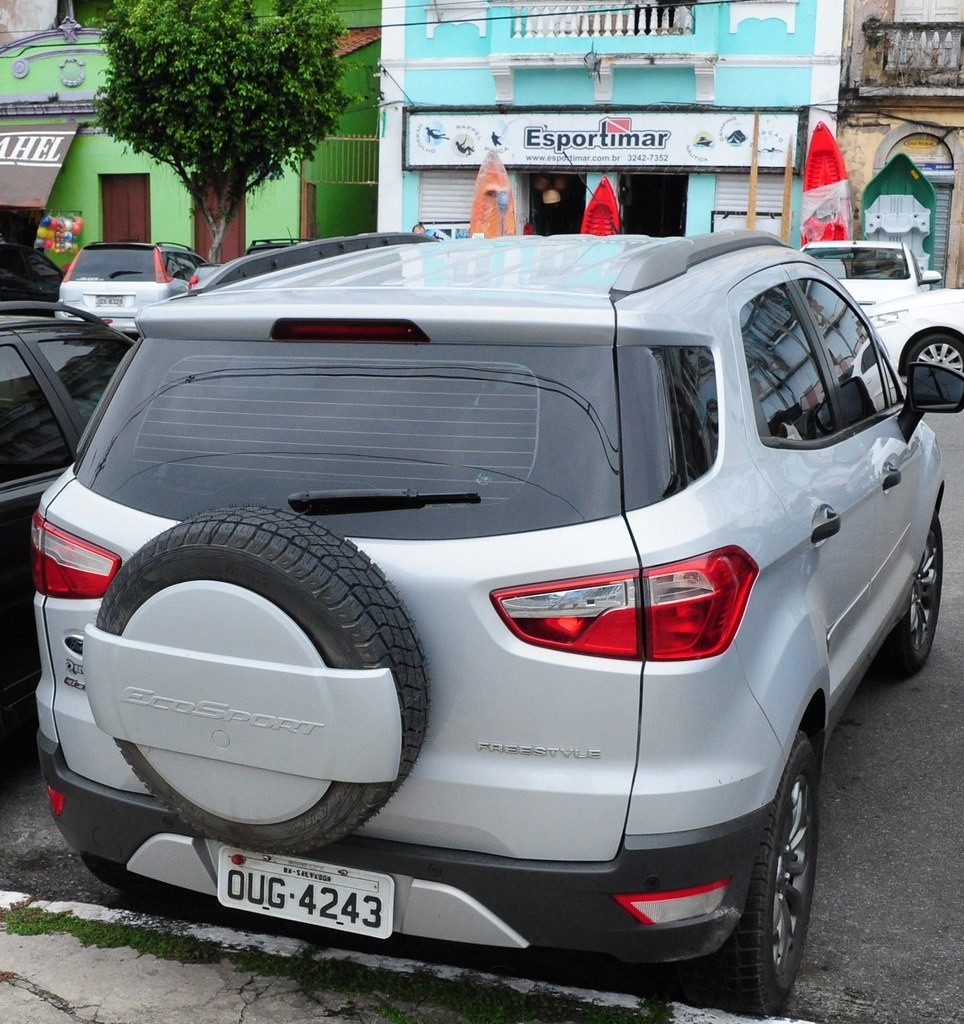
412,224,451,242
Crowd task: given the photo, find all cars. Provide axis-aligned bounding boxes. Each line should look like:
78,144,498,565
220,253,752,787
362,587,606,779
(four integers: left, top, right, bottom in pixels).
862,285,964,383
797,240,944,309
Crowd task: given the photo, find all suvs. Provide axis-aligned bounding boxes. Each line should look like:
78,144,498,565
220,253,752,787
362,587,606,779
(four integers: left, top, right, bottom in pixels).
27,230,948,1014
53,241,208,338
0,240,67,316
0,299,141,715
